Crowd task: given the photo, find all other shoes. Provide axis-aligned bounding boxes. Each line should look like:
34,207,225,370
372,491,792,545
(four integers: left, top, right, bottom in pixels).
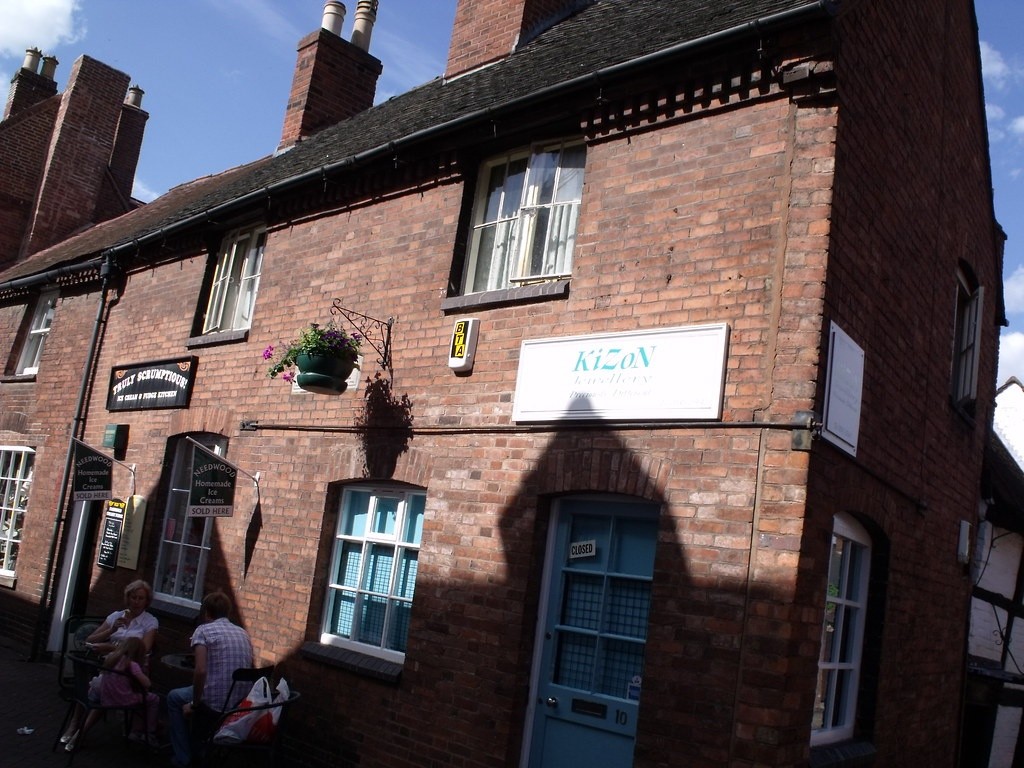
60,732,71,743
170,756,182,768
64,743,75,751
141,733,160,749
128,729,145,745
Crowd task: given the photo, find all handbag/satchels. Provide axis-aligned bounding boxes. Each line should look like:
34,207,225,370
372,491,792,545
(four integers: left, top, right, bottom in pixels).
205,677,289,746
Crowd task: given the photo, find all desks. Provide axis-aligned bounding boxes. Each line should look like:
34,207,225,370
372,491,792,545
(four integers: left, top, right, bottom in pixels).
161,653,195,687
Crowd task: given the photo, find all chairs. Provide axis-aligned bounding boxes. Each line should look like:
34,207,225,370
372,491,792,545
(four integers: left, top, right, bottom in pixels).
189,667,270,741
52,649,151,768
203,691,301,768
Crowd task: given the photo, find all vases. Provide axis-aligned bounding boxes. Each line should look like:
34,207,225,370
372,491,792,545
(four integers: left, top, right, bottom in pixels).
296,354,354,396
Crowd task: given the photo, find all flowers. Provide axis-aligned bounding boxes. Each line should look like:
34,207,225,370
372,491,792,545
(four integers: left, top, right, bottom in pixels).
262,319,364,384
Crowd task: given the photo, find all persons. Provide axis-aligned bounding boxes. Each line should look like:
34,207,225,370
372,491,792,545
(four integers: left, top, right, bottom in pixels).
161,592,253,768
99,638,159,748
58,579,159,753
